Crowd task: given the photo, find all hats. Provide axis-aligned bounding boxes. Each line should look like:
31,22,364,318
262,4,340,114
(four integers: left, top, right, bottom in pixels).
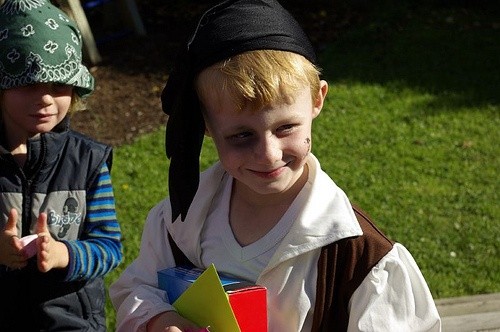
1,0,95,104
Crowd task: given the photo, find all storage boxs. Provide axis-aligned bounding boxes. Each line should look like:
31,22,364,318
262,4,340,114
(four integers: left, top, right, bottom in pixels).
157,265,269,332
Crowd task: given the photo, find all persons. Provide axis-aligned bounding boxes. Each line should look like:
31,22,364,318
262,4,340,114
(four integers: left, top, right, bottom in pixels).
109,0,441,332
0,0,122,332
